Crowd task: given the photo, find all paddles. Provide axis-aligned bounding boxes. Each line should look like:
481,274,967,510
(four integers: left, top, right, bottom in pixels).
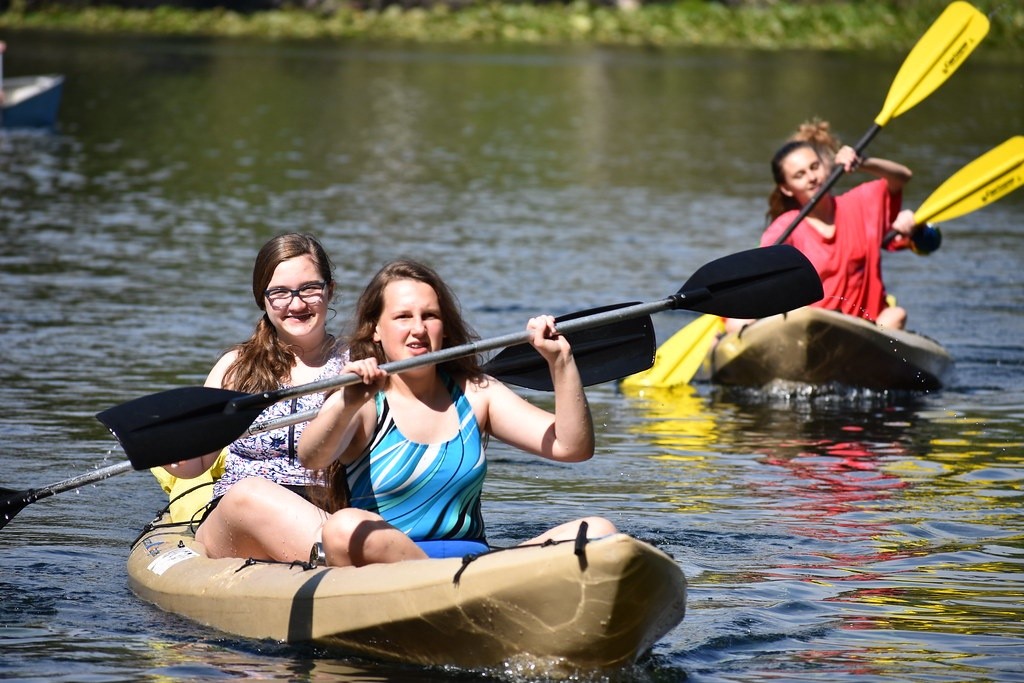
95,243,825,472
879,134,1024,249
0,300,658,531
619,1,991,388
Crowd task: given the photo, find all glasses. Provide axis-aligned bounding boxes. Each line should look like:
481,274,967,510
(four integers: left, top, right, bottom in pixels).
263,281,328,311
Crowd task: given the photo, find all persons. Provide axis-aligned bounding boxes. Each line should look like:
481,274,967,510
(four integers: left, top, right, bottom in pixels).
165,235,351,566
710,118,942,356
298,258,616,567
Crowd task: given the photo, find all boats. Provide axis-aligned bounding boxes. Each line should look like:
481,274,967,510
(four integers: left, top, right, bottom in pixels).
693,303,950,393
0,74,66,129
125,501,689,683
